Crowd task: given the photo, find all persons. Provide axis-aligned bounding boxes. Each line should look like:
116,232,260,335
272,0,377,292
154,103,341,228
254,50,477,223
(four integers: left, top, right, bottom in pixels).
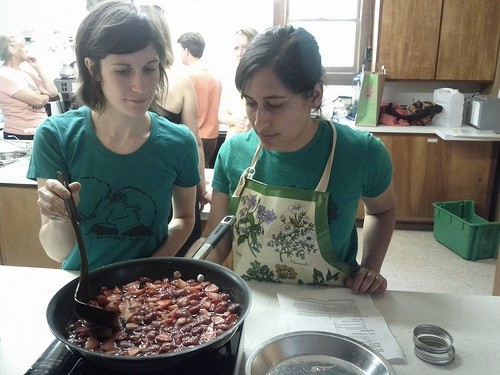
150,6,213,256
218,27,259,142
183,25,397,294
1,34,58,140
177,32,222,168
26,0,201,269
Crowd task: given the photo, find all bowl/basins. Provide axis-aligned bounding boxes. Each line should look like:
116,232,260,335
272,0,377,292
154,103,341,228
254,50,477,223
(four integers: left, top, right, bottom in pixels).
245,331,397,375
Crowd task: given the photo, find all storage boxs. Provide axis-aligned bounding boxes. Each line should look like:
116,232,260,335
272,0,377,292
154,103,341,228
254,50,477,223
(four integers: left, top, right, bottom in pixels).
432,199,500,261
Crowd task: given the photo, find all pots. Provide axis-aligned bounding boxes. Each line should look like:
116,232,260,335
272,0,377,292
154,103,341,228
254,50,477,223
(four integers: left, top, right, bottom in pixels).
45,213,252,375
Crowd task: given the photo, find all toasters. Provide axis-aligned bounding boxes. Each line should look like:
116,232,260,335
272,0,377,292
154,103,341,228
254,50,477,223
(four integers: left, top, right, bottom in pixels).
467,92,500,129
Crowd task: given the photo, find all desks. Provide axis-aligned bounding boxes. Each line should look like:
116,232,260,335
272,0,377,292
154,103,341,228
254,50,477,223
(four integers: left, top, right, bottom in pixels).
0,138,500,374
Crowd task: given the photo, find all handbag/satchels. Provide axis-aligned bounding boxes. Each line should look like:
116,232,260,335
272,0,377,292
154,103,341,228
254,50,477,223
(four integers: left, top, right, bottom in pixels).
379,100,443,126
354,70,394,127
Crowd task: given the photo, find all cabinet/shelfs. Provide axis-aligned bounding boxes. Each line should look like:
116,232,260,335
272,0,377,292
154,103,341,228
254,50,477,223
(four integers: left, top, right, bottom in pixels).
356,125,500,223
372,0,500,80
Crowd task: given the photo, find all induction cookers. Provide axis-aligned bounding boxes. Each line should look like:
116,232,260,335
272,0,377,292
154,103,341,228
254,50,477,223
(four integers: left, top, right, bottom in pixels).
23,320,245,375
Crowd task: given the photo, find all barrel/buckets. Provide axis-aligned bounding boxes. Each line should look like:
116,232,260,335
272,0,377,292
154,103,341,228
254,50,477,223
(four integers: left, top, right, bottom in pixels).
432,88,464,127
